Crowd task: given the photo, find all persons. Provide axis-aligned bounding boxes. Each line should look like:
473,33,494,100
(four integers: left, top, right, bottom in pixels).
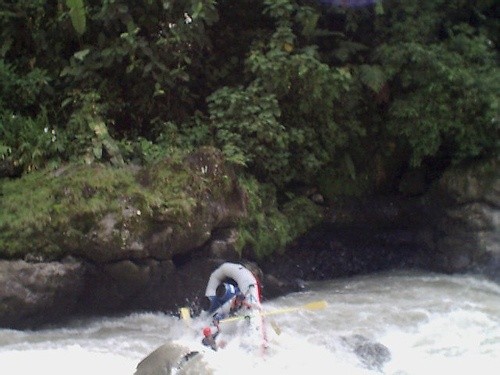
227,293,261,320
200,322,222,352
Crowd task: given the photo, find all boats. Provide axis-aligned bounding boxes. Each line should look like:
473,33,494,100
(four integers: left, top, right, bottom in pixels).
180,263,263,352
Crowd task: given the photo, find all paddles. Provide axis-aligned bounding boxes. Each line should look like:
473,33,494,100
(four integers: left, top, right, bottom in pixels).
215,300,327,324
